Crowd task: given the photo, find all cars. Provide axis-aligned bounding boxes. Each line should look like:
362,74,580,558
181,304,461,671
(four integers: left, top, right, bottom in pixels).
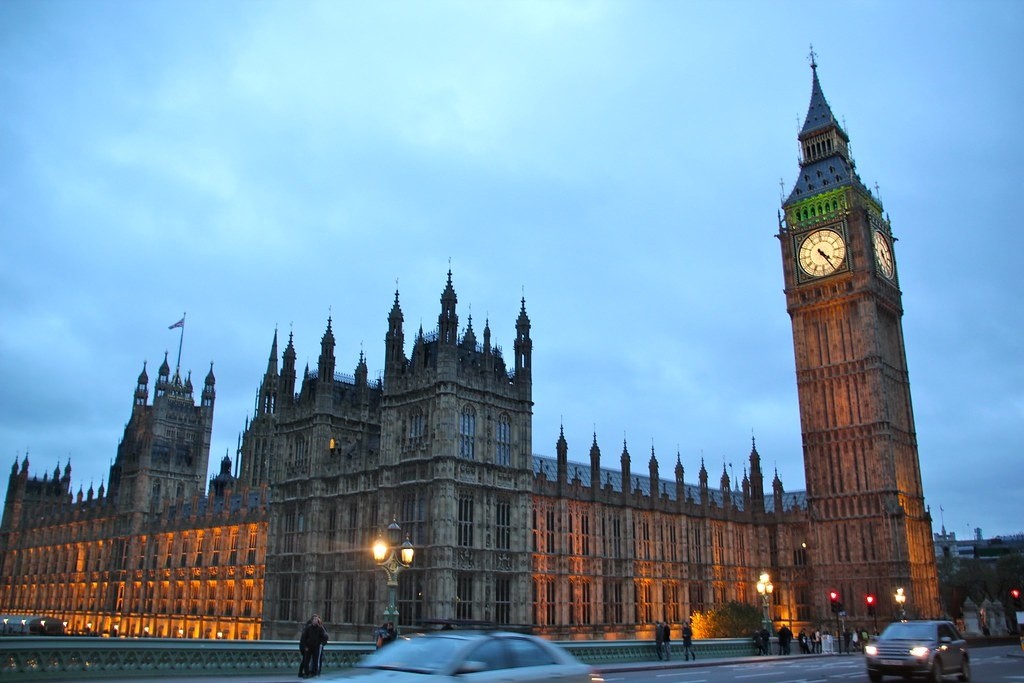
863,619,971,682
300,630,605,683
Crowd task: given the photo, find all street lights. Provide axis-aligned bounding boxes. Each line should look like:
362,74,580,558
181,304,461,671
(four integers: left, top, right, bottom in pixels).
39,618,46,635
86,621,91,636
895,587,906,621
2,618,8,634
113,623,120,637
757,570,774,655
61,619,68,635
19,619,27,634
371,513,415,634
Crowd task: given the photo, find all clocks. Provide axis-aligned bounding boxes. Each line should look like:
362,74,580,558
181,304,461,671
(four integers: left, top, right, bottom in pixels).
793,221,849,285
869,217,901,291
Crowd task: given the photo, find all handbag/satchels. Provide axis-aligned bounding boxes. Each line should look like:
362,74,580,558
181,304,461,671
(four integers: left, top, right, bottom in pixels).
377,635,383,648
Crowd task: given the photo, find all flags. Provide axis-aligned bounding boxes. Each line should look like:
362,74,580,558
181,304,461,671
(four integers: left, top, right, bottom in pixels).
168,317,184,330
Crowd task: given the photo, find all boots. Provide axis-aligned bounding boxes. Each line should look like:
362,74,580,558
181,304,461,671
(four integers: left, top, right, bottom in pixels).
685,653,689,661
692,652,696,661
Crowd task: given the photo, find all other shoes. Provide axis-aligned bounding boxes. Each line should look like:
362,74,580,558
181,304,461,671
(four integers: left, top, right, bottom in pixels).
665,658,670,661
657,657,663,661
298,673,320,679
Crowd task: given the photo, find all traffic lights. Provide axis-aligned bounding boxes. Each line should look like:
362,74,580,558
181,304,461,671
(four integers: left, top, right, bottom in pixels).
867,597,874,616
829,592,838,612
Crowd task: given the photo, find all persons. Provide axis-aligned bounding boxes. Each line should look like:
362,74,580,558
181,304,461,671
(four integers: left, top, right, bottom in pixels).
374,621,399,652
753,628,771,656
298,614,327,677
777,624,793,655
654,621,672,661
682,621,696,661
798,625,870,655
